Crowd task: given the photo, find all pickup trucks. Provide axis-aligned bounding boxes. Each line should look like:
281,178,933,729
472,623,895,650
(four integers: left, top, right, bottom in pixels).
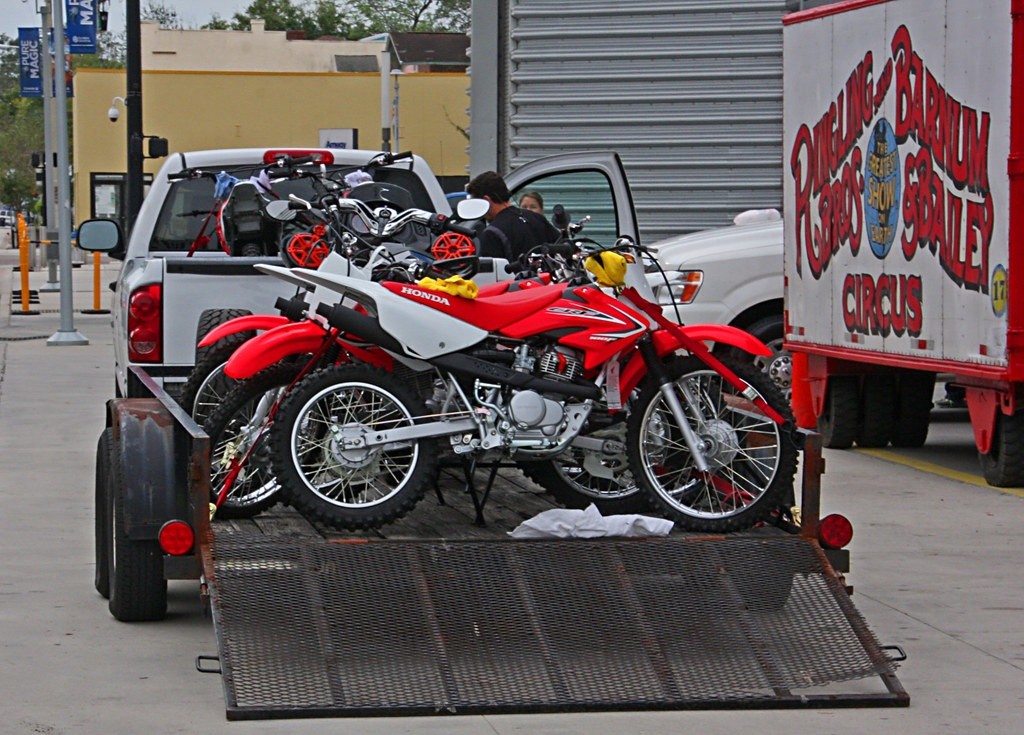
75,147,640,397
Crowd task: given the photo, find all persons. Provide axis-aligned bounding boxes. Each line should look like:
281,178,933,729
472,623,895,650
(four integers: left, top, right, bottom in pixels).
467,171,562,270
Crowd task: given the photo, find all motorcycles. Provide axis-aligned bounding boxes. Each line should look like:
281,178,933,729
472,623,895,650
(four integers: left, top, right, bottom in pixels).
177,154,800,531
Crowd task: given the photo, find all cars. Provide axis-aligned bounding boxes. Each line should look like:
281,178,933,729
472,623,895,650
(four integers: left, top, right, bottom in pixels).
642,209,795,416
0,210,36,226
